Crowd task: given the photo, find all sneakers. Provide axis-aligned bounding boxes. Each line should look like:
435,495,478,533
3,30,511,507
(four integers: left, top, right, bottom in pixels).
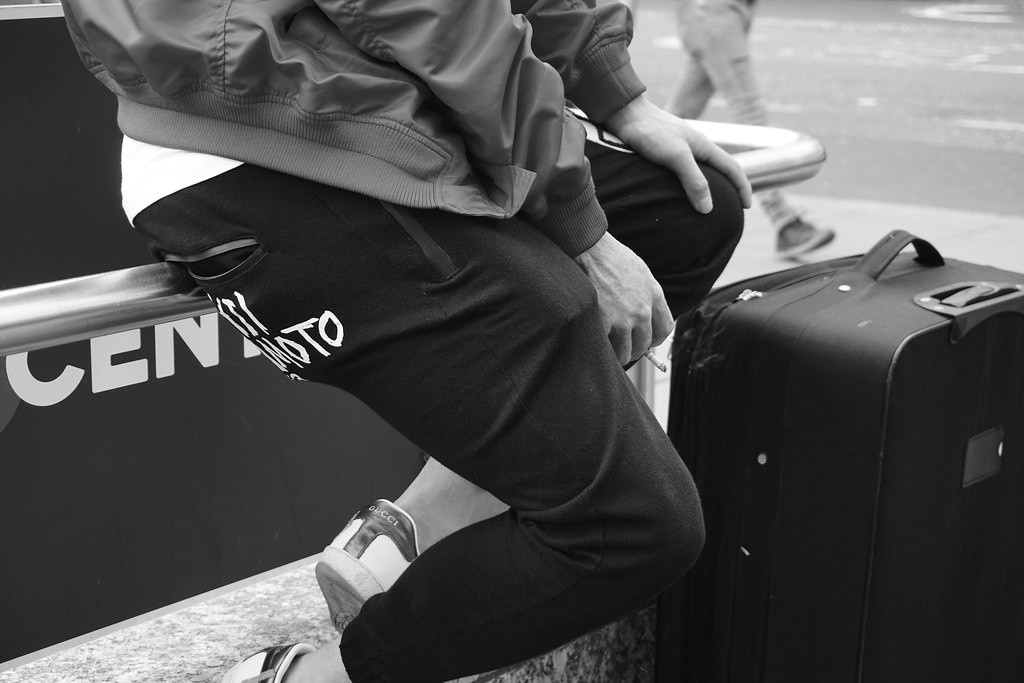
316,499,477,683
223,643,316,683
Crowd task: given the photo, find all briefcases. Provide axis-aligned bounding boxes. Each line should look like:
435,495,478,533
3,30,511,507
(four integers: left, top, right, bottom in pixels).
652,228,1024,683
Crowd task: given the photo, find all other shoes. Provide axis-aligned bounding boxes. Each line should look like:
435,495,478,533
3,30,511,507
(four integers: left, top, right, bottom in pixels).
778,221,835,254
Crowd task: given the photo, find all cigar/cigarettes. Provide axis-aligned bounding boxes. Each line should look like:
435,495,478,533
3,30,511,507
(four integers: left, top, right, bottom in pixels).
643,350,667,373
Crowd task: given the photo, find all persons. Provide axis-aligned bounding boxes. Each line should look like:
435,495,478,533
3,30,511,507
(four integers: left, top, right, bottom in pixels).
663,0,836,257
60,0,754,683
